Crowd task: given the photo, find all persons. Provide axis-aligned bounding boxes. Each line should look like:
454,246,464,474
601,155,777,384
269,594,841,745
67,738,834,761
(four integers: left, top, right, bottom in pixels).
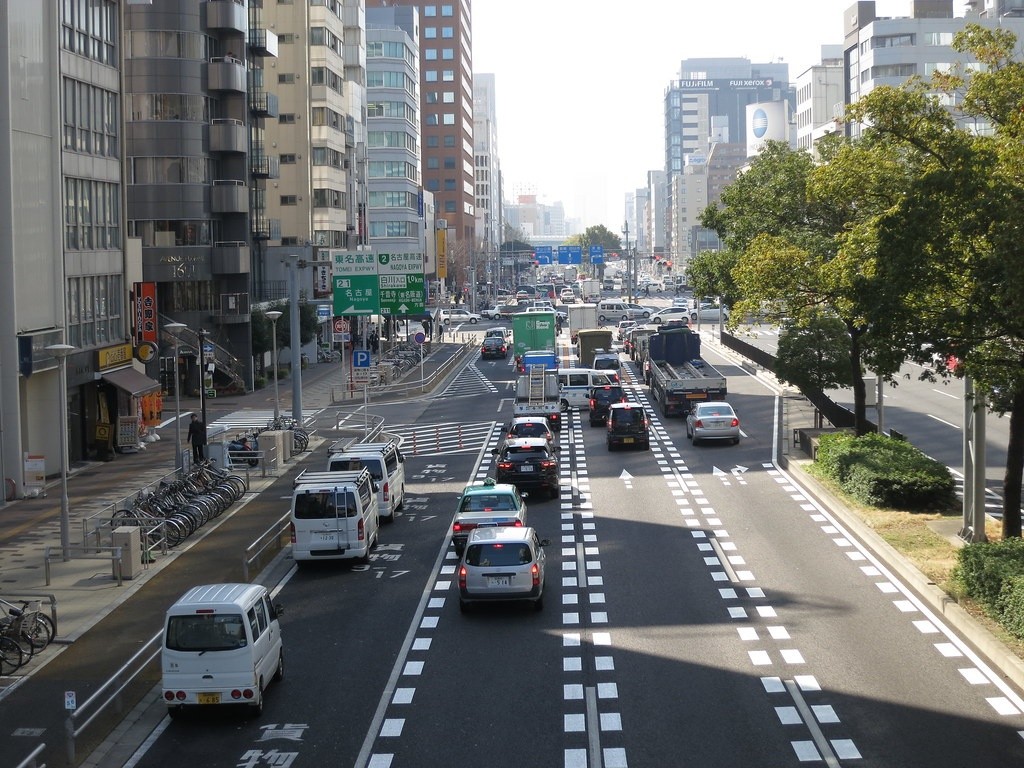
369,330,379,353
187,413,207,463
422,320,429,338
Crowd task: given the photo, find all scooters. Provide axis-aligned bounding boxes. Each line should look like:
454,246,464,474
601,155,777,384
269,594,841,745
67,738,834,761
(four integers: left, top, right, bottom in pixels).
226,434,259,466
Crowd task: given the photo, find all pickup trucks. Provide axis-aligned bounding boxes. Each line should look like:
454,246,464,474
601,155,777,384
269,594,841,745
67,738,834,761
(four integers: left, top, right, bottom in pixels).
482,306,505,320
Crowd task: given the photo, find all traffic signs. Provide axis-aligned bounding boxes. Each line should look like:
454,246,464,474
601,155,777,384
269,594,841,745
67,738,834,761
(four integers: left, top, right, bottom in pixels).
331,252,425,316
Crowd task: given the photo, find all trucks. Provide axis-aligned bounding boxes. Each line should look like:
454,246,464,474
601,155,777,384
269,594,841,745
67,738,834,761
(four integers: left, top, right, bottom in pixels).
641,322,728,418
511,372,563,433
580,279,600,301
512,311,559,373
568,303,614,368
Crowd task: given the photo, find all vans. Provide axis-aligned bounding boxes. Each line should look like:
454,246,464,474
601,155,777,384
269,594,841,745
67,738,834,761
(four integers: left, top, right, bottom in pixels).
596,301,647,361
545,369,612,411
291,437,402,567
162,583,284,717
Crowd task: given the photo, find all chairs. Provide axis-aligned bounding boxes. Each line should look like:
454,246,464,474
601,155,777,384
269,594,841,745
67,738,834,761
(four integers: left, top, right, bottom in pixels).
497,502,511,507
466,503,482,508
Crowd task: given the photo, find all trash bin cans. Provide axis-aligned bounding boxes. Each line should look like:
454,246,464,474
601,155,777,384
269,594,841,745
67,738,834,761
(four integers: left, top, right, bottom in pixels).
208,442,229,469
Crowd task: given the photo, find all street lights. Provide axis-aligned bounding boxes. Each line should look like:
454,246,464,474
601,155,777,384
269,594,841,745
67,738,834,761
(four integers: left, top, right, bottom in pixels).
164,322,188,474
266,310,284,418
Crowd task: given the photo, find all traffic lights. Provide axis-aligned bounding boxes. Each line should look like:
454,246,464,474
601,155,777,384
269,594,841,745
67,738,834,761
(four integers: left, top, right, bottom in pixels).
657,261,672,271
650,256,660,260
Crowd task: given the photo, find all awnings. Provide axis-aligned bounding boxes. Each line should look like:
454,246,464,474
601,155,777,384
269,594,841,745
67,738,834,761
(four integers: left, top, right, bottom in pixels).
99,364,161,398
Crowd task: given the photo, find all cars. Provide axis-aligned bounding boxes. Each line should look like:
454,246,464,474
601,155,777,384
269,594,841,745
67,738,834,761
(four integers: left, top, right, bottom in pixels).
499,278,574,310
629,298,730,325
482,326,510,359
452,484,550,612
502,417,558,441
684,402,740,446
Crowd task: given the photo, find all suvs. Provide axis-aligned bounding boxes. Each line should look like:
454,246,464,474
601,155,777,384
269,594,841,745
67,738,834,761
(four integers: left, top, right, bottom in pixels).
586,386,650,451
439,308,481,325
491,439,558,496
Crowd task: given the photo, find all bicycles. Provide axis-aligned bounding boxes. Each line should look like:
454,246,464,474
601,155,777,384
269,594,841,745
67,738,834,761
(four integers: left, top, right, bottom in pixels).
251,417,308,455
368,346,428,386
110,457,246,547
0,599,55,677
